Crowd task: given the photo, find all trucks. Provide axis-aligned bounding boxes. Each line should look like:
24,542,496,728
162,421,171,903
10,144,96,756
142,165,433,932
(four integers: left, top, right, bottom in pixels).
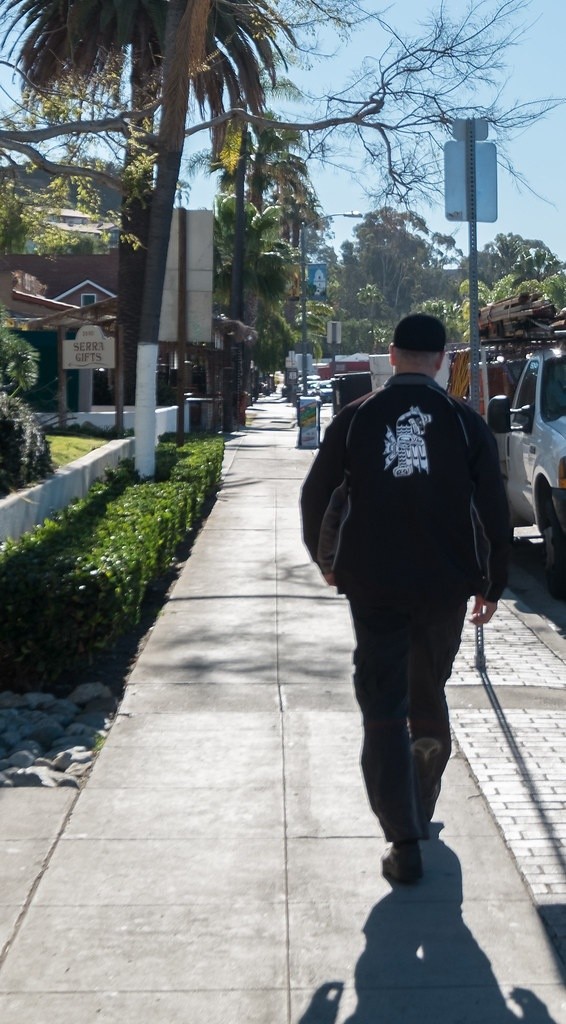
445,339,566,601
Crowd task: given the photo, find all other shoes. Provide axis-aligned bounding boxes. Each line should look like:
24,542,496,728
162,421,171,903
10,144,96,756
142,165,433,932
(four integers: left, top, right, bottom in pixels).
381,841,423,880
423,777,443,822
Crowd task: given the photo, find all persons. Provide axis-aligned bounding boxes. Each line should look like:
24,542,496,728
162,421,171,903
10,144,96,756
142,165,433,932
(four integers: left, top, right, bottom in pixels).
300,313,515,884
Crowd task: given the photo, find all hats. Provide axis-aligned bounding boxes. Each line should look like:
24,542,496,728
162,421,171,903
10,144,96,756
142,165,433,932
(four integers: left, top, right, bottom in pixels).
395,315,446,352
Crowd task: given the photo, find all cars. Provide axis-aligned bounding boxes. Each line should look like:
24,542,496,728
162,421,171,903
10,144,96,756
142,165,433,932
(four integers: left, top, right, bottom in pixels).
297,375,333,403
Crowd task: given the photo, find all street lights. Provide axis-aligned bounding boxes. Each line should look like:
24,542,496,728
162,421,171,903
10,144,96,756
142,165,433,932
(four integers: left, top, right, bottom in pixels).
302,211,362,397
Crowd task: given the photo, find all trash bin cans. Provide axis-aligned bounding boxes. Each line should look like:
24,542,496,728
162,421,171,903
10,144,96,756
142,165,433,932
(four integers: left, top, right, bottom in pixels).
330,371,372,421
